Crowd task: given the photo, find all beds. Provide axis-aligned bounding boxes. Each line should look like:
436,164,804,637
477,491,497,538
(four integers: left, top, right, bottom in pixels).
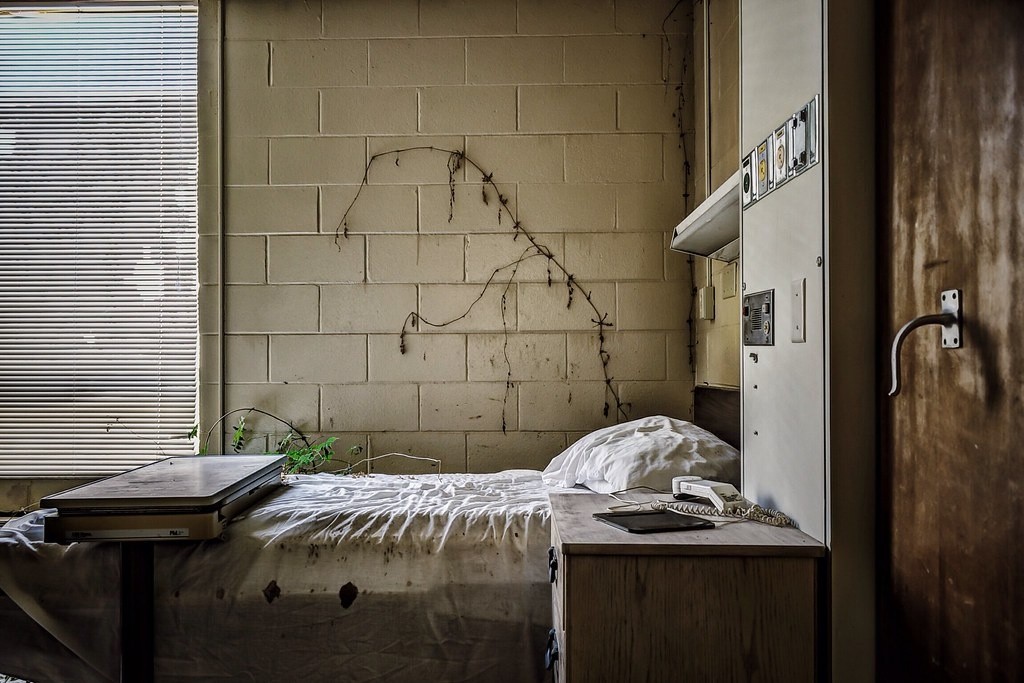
0,381,743,683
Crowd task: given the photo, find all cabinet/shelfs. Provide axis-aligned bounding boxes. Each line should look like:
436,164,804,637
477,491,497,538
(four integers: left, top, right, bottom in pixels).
548,494,826,683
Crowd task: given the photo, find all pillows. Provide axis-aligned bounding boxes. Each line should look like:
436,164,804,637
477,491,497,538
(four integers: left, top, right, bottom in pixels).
541,416,741,497
